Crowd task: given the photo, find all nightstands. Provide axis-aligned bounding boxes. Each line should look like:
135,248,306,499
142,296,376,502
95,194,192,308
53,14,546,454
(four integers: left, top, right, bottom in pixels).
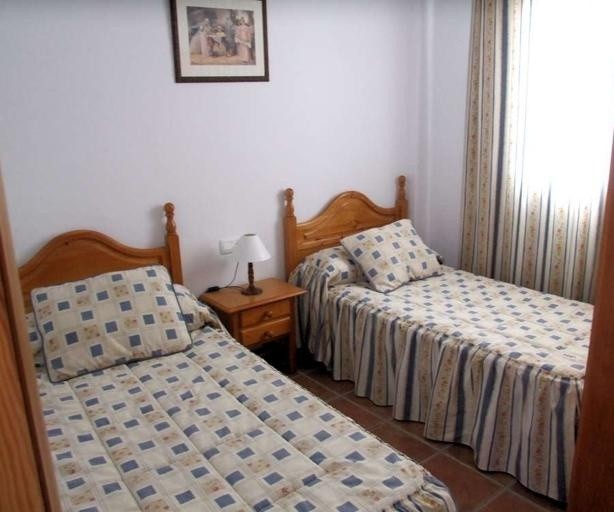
200,278,306,375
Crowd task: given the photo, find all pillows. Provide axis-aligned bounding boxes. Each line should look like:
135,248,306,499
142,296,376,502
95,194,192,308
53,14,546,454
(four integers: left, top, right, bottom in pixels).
339,217,443,292
30,265,192,383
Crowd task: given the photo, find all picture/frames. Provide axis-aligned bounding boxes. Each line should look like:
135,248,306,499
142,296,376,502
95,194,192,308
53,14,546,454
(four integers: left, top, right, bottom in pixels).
170,0,270,84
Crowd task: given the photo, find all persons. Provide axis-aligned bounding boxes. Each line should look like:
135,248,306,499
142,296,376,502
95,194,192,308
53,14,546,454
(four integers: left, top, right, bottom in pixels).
190,12,253,63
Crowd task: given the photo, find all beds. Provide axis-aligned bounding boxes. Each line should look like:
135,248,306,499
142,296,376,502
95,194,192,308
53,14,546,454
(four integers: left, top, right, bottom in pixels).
16,203,458,511
283,175,596,503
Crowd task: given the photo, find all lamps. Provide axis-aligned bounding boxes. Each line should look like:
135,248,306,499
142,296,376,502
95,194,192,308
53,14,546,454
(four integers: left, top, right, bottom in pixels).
233,234,271,296
